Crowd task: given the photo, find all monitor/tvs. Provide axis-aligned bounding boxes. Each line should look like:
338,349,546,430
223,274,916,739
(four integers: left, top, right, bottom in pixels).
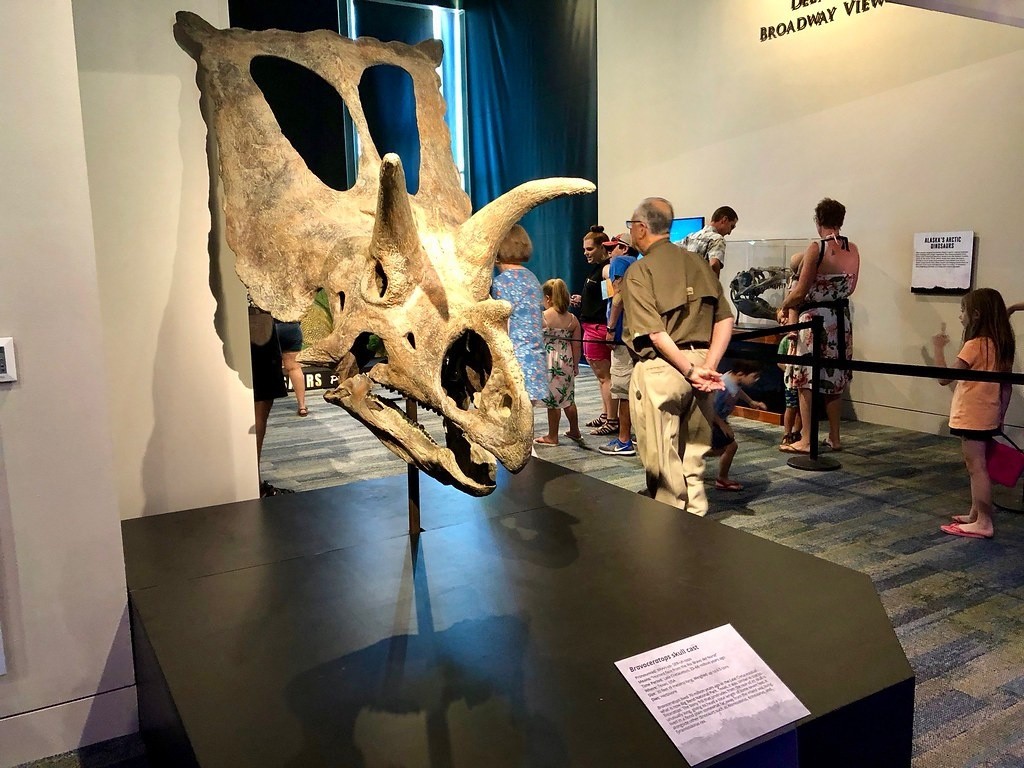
638,217,705,261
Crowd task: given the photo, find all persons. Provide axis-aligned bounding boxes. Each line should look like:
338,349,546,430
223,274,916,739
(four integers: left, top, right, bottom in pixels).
571,225,620,436
673,206,738,280
490,223,550,407
247,287,294,500
776,199,859,452
705,348,768,490
932,288,1016,539
598,233,639,455
274,319,308,417
533,278,583,446
620,198,736,516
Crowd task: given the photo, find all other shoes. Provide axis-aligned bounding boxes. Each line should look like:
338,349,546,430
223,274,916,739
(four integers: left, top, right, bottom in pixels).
259,481,295,497
783,434,794,446
791,432,802,443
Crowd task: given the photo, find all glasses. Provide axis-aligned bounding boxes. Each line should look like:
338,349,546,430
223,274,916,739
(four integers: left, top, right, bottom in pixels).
611,236,630,246
627,220,643,230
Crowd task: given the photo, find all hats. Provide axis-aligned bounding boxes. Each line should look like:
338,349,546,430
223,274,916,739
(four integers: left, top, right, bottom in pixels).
601,232,639,251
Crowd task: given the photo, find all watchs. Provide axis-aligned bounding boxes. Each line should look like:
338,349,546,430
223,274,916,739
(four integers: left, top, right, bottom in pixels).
606,326,616,333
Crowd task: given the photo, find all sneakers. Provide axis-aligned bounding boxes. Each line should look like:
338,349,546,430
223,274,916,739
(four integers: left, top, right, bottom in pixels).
599,439,636,455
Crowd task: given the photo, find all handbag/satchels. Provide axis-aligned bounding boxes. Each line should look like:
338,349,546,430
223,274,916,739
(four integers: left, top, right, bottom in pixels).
985,437,1023,487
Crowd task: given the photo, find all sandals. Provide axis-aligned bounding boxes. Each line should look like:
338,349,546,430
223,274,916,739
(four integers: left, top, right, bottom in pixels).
586,414,607,427
590,418,621,436
298,407,308,417
716,478,745,492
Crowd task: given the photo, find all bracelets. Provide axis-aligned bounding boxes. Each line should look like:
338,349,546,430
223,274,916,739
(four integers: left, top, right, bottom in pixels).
685,363,694,378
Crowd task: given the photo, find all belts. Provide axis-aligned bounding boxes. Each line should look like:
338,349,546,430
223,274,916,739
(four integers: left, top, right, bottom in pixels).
676,341,709,350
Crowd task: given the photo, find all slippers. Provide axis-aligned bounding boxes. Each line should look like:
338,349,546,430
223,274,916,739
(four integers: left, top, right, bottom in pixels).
533,436,559,447
940,521,986,540
822,437,842,450
952,515,971,525
563,430,584,441
782,446,810,454
